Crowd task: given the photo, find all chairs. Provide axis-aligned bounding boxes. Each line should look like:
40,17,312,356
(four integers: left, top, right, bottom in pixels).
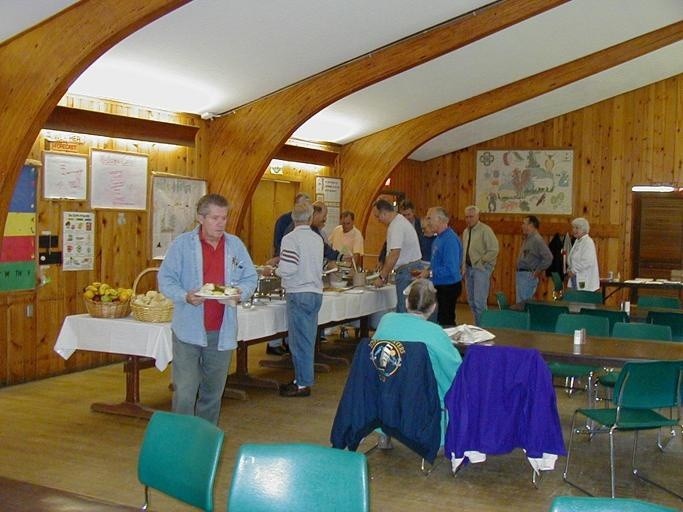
551,271,564,303
562,361,683,497
226,441,371,512
548,494,679,511
135,409,225,510
524,302,570,333
548,313,610,432
637,294,682,310
580,307,631,337
449,344,544,490
589,321,672,443
359,343,446,483
562,290,603,305
478,309,530,332
646,311,682,342
496,291,510,310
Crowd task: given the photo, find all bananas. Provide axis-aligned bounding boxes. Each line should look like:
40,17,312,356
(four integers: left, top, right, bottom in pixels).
84,281,118,297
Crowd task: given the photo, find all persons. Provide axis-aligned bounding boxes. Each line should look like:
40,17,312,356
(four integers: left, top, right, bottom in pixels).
460,206,499,326
371,198,424,312
278,200,358,355
418,215,436,266
416,207,463,327
515,215,553,303
370,278,461,448
265,193,308,355
328,210,363,339
378,200,420,265
157,194,258,428
259,202,324,397
564,217,599,292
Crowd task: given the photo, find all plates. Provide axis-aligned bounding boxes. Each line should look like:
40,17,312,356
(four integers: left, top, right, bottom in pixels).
254,268,266,270
194,293,240,299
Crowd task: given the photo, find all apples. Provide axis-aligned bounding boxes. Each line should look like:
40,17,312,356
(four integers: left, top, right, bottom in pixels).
101,294,112,302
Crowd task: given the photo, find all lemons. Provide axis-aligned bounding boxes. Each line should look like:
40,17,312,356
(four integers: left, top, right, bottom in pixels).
118,287,133,302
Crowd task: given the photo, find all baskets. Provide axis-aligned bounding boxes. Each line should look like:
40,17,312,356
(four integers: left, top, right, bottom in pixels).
129,267,174,323
84,295,132,319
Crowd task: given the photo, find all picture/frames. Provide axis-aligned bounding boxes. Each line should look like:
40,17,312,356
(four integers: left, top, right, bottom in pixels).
88,146,151,214
41,150,90,203
472,144,578,219
313,175,343,240
146,172,210,263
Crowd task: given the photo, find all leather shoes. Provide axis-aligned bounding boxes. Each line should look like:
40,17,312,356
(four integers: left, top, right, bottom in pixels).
266,343,285,355
281,384,311,397
279,382,294,388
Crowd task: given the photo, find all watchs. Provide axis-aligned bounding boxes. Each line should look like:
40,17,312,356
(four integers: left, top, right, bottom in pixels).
270,269,274,277
379,274,386,283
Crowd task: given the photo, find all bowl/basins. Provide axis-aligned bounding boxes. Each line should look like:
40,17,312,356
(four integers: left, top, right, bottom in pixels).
329,280,349,287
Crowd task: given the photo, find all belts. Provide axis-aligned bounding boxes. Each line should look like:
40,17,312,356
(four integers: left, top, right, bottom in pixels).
517,268,534,272
